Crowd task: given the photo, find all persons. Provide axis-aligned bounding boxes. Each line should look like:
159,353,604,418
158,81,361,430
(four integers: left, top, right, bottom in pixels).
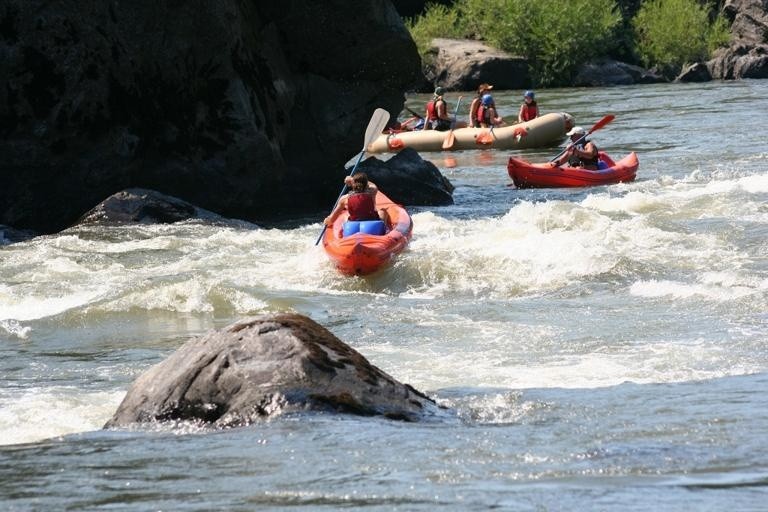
544,125,599,171
389,112,419,133
477,94,508,128
323,172,393,233
420,86,467,131
519,89,539,123
469,83,494,128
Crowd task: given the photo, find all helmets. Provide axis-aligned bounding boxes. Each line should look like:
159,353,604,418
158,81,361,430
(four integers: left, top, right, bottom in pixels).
482,94,493,105
524,90,534,98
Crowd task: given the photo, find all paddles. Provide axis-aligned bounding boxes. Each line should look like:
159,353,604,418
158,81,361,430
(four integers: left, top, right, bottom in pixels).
442,95,462,149
313,108,391,246
476,124,494,145
548,115,615,163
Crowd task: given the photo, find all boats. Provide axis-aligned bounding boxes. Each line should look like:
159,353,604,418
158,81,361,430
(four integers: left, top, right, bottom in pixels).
322,183,411,280
506,152,641,189
367,113,575,154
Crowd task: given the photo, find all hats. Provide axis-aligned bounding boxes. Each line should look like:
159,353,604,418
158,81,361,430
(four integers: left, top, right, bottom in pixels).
479,82,494,94
564,127,587,137
435,87,448,96
